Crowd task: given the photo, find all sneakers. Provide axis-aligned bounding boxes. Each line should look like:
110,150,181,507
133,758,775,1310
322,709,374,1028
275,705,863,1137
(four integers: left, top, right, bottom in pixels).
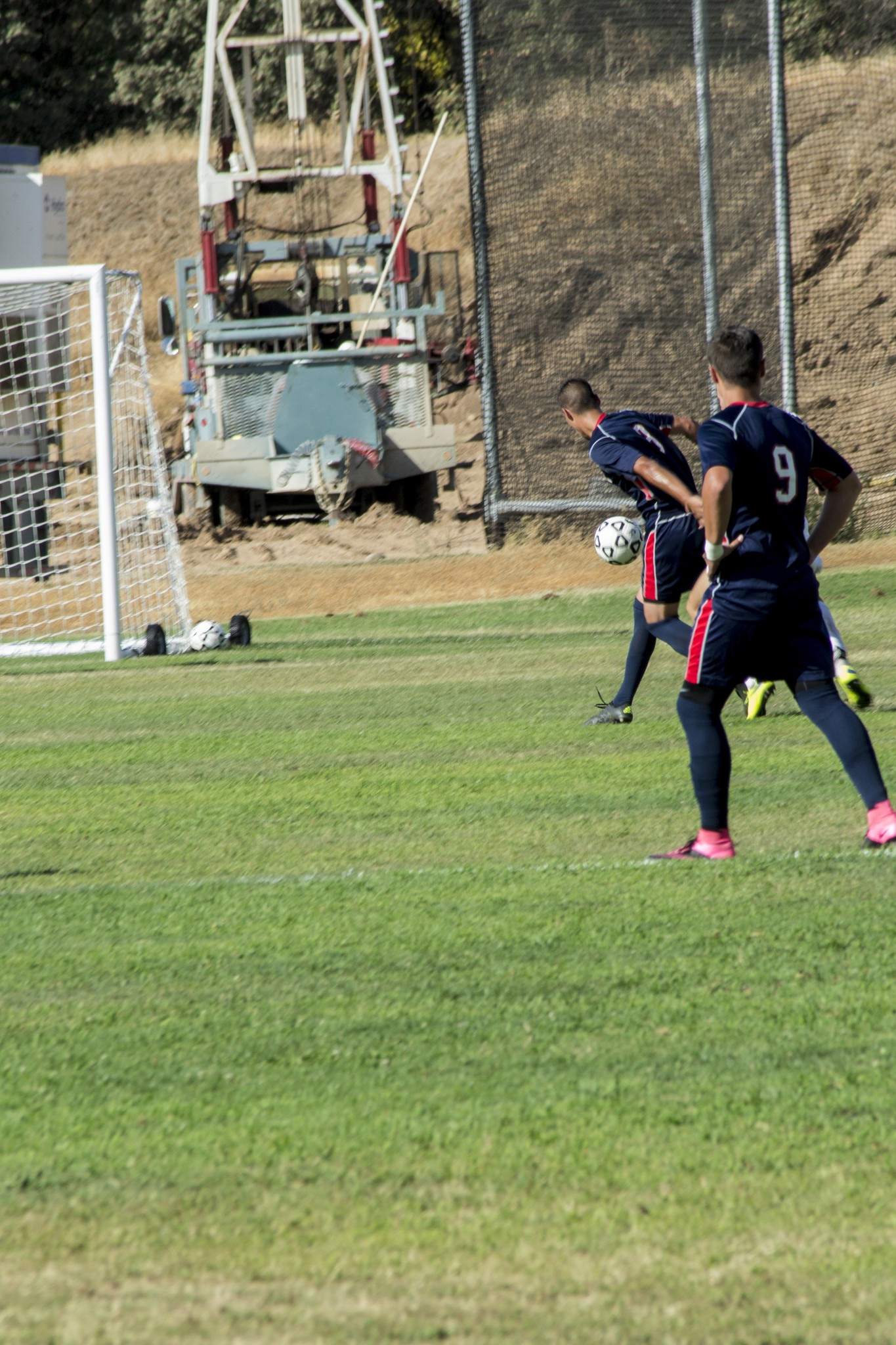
834,664,872,712
582,701,634,723
644,828,736,861
860,799,896,850
743,679,777,720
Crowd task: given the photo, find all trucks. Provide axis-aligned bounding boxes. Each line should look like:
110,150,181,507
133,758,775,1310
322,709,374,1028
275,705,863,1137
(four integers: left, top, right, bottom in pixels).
151,271,460,533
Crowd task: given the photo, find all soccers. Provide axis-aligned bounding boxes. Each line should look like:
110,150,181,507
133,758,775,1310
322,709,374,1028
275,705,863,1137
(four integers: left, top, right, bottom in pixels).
190,620,226,651
594,516,643,566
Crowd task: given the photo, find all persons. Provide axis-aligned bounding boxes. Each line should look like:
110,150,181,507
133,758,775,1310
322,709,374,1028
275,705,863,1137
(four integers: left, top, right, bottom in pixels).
558,377,776,725
645,330,896,862
743,516,872,721
